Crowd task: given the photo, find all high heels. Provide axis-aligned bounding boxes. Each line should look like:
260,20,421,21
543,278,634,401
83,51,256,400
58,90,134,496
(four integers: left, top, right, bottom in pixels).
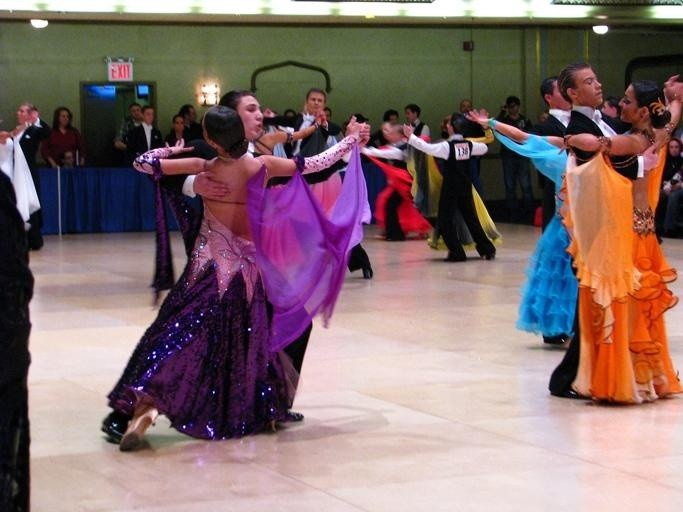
119,406,158,450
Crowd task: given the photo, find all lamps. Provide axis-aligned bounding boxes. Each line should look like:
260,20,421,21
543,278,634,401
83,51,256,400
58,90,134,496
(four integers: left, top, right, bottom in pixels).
31,19,48,29
593,26,609,35
198,83,221,107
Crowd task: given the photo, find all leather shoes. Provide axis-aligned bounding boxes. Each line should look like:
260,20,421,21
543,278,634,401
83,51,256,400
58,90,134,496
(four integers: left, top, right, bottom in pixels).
101,412,127,440
363,265,372,279
550,381,589,398
484,252,495,260
384,236,404,241
443,255,466,262
543,334,566,344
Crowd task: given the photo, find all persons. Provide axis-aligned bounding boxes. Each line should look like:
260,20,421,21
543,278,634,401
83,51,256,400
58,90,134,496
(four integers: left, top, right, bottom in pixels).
0,102,87,251
465,63,682,406
113,101,200,168
263,87,502,262
1,169,34,512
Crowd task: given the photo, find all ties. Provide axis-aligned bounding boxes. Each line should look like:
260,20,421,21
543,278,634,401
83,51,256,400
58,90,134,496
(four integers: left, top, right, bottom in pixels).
591,110,616,137
